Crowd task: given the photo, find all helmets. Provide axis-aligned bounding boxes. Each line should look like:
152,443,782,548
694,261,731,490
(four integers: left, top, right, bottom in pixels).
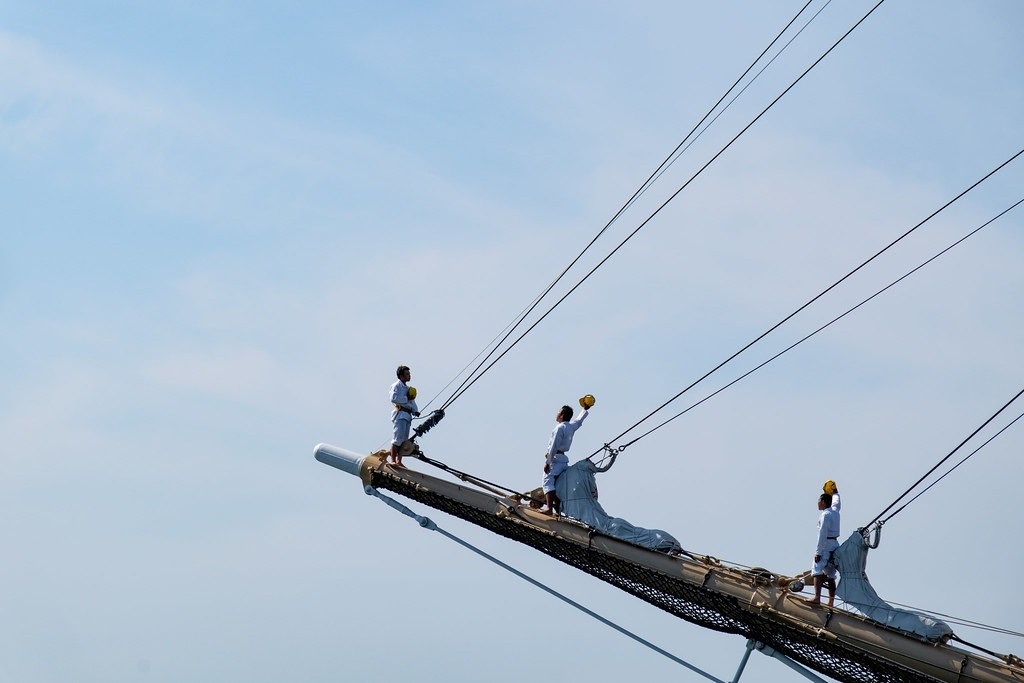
823,480,838,496
579,395,595,410
407,387,416,400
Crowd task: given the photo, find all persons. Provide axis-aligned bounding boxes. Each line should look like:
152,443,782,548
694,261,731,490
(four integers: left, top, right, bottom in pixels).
389,366,420,468
536,404,589,516
803,492,841,607
